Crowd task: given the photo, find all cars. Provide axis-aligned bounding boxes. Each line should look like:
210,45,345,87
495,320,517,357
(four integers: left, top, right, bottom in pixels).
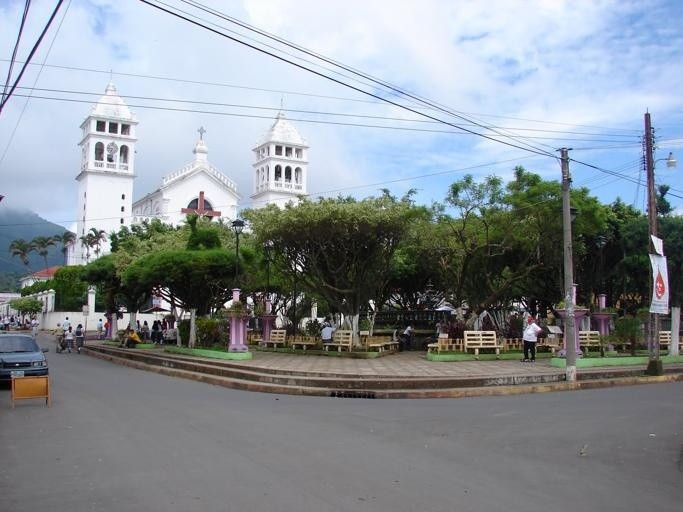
0,332,49,387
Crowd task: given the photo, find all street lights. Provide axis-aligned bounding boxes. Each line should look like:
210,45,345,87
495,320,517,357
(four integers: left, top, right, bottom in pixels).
231,218,245,288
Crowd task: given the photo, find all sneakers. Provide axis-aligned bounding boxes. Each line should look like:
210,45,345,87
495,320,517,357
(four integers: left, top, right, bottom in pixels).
531,359,534,363
521,359,529,362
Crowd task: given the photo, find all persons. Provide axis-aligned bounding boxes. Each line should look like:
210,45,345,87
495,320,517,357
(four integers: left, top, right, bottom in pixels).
403,321,415,351
434,319,448,337
0,313,179,354
519,316,543,362
392,324,407,351
320,321,336,351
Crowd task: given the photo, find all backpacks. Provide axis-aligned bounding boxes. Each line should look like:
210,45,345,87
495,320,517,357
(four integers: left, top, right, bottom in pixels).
76,328,82,336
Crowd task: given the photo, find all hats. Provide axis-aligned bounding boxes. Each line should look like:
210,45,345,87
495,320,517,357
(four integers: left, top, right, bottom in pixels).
323,322,330,326
527,318,533,323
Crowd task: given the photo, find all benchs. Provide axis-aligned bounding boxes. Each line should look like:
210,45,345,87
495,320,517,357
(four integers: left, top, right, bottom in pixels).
658,330,683,352
291,336,317,350
463,330,505,360
427,339,441,356
323,329,353,352
365,335,400,352
577,330,604,358
263,330,287,352
